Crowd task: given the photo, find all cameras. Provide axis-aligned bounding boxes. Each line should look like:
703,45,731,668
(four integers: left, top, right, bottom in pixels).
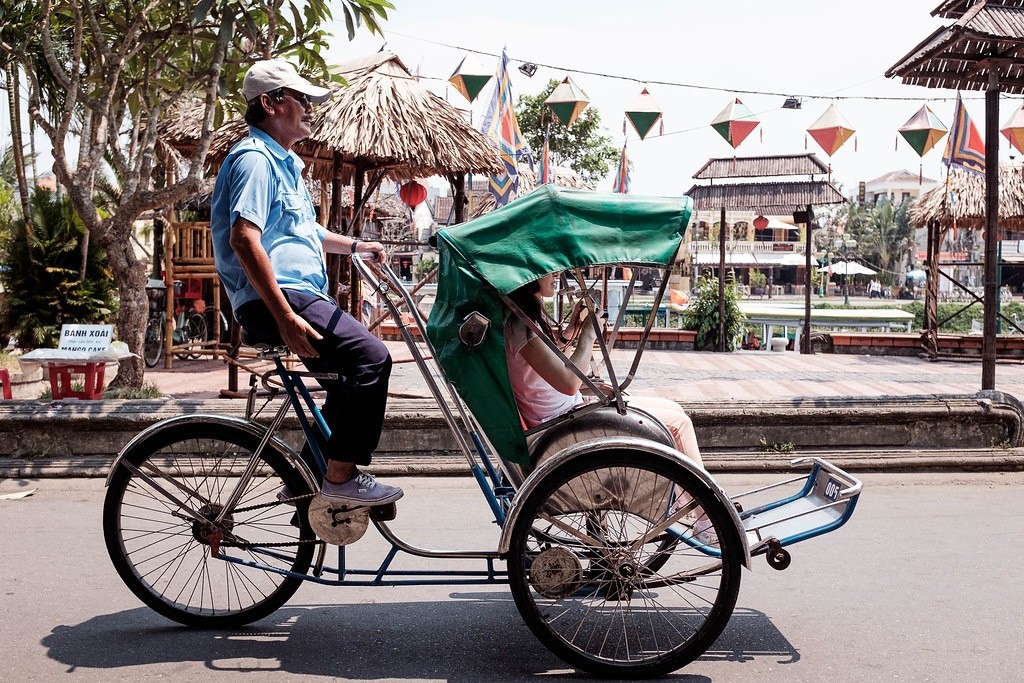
578,305,609,327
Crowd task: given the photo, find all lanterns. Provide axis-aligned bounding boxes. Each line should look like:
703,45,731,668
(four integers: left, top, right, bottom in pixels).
399,179,428,214
752,214,768,237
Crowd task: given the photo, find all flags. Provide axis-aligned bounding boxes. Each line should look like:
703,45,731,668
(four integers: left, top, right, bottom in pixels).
942,95,984,177
479,52,532,204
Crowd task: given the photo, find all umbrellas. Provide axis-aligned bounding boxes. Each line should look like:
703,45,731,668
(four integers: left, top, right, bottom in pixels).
907,269,927,290
817,259,878,298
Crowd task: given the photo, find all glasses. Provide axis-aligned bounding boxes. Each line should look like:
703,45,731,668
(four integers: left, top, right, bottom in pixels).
294,93,312,108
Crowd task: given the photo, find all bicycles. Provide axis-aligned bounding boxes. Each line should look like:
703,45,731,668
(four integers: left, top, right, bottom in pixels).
140,279,230,369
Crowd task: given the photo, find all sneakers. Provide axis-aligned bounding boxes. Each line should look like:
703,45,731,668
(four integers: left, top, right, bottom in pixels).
670,502,698,527
278,486,299,507
321,470,404,507
692,524,722,548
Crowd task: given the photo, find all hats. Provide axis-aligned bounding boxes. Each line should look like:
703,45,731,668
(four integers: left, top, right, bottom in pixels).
243,59,331,103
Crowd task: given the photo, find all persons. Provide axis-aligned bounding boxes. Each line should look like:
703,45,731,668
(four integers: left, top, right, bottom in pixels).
211,59,407,511
500,269,721,549
867,278,883,299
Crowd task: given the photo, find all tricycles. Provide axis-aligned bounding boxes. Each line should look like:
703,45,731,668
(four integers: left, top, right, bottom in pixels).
100,185,863,678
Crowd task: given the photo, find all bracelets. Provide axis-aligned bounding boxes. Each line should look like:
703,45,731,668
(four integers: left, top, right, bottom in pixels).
350,239,362,254
558,330,570,345
